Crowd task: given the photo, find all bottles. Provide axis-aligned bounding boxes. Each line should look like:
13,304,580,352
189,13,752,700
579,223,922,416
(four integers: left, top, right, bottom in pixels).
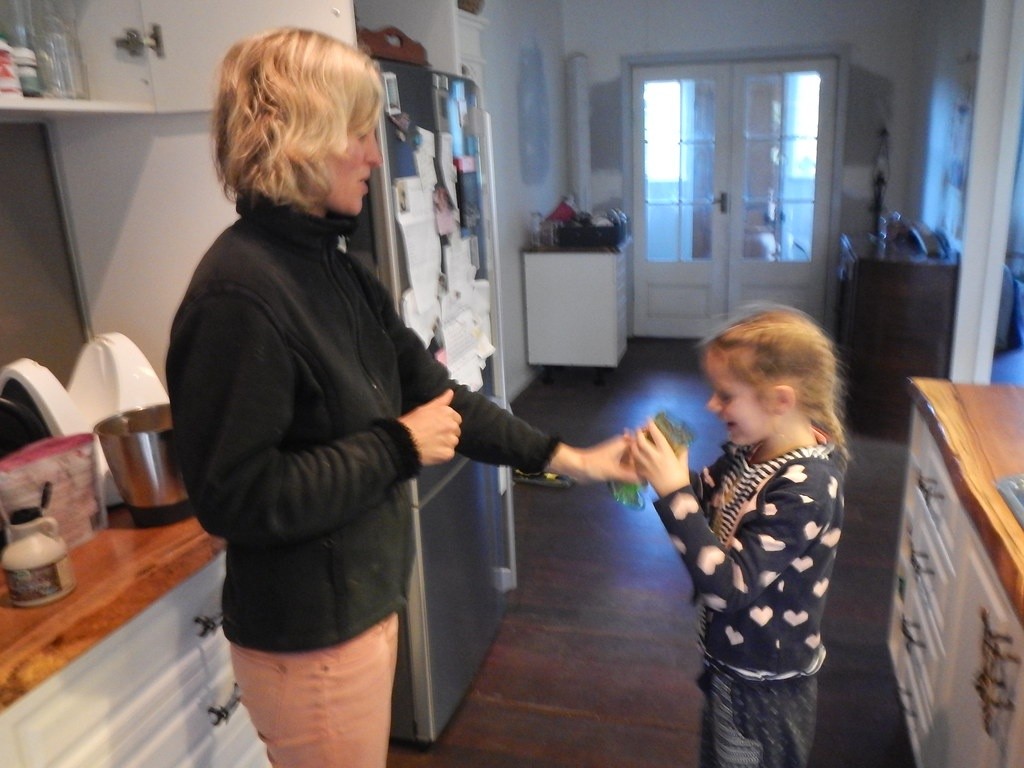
1,507,78,607
529,211,544,248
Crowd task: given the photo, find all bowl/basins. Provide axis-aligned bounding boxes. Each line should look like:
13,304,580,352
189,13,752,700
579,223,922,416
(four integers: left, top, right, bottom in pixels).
96,403,191,526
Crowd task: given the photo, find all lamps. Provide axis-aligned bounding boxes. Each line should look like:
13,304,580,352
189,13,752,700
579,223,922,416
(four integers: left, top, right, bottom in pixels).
865,127,890,232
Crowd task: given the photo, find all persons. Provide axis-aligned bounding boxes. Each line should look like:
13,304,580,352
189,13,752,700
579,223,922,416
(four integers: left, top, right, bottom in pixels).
165,28,847,768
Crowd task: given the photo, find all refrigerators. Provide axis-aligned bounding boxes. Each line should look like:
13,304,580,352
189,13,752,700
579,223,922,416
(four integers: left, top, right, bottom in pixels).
354,51,516,748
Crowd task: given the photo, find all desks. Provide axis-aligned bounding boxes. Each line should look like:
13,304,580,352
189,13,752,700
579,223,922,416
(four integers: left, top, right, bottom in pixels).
887,409,1024,768
0,550,274,768
830,233,958,439
523,238,632,381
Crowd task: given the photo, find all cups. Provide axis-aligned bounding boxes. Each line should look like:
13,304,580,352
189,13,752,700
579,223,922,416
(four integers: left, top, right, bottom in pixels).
609,410,694,511
